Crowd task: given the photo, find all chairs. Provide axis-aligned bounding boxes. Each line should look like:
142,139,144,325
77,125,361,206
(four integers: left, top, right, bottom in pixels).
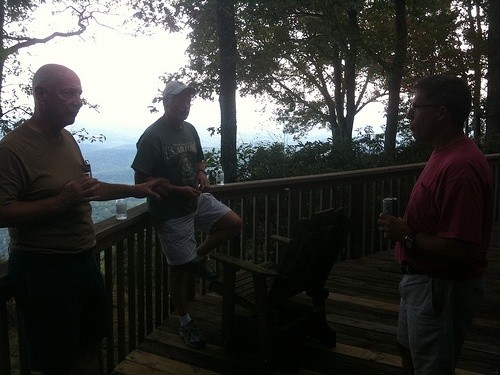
211,204,350,350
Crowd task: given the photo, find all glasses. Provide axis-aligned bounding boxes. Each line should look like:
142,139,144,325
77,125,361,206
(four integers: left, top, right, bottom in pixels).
410,102,436,110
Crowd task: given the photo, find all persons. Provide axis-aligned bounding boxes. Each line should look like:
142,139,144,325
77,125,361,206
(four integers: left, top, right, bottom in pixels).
0,64,166,374
130,81,243,349
376,75,500,375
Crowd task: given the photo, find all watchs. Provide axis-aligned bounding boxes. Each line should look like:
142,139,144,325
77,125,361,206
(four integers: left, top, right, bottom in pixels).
403,230,417,249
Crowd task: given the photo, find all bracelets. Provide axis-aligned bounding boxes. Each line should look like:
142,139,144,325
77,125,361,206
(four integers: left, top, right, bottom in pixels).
197,168,208,176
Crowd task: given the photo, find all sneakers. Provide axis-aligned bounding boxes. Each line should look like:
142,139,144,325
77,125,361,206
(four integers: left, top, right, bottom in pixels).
180,323,208,350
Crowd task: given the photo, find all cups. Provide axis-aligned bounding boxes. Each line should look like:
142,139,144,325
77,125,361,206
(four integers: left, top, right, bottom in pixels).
116,202,128,220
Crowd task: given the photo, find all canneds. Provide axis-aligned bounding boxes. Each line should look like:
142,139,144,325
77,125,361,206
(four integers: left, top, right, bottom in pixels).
382,197,400,239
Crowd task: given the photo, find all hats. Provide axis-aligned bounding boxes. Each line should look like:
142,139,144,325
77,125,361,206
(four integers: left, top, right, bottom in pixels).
162,84,197,98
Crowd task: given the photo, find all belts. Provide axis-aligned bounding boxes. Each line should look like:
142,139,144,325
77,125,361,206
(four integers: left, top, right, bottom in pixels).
401,265,449,278
